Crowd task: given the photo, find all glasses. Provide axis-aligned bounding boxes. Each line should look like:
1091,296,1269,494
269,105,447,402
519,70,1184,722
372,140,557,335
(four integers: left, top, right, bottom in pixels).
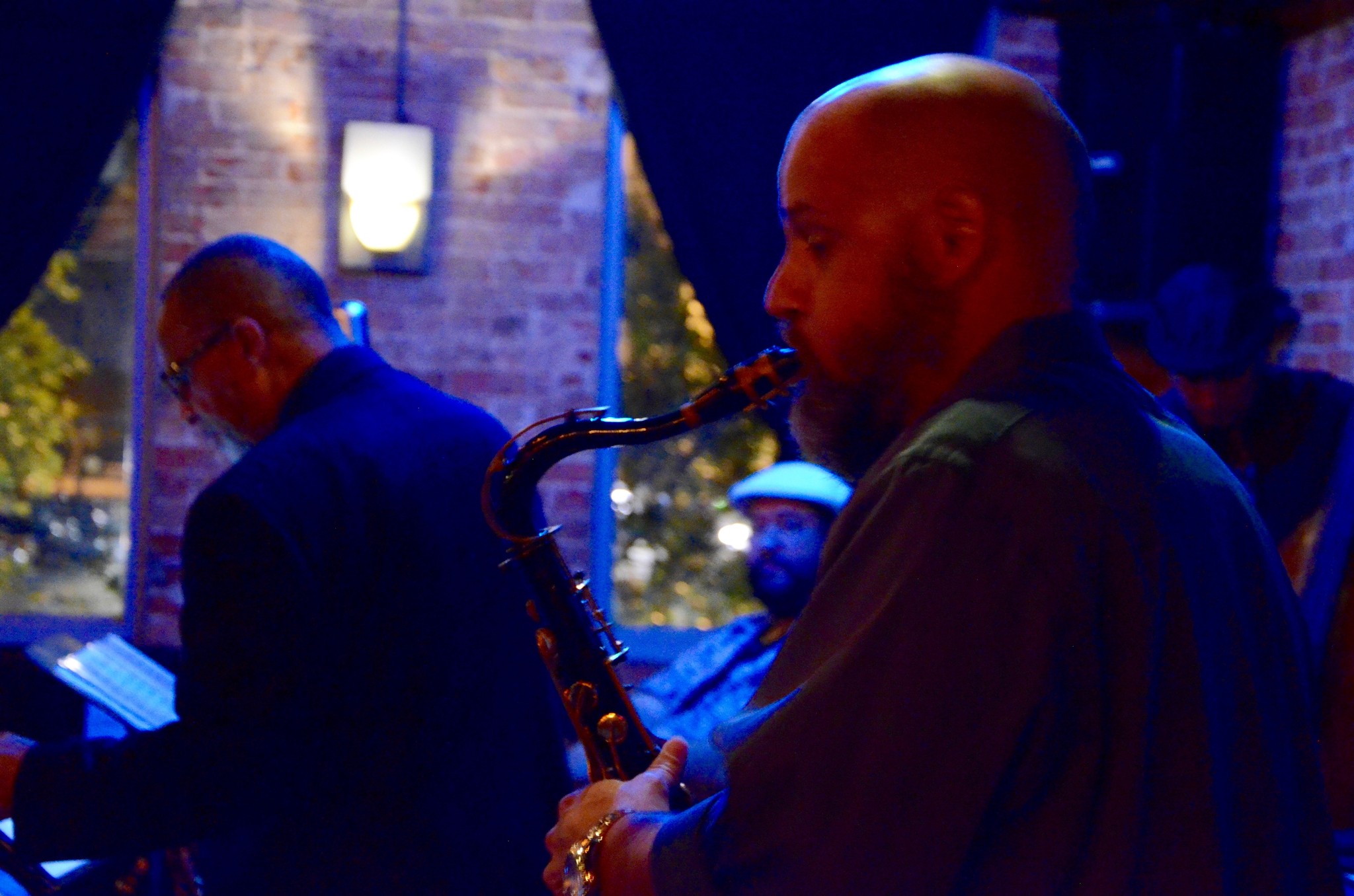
159,314,240,405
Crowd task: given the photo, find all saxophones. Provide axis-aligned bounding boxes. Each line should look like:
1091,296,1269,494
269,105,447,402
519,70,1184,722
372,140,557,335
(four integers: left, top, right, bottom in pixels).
479,345,809,817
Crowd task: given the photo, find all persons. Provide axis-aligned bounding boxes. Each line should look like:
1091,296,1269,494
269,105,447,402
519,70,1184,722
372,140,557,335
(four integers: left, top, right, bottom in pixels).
1,232,577,896
537,46,1346,896
558,459,852,790
1137,256,1350,594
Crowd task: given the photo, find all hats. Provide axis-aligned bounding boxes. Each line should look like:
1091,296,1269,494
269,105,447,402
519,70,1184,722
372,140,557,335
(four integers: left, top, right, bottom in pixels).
1147,266,1261,382
727,459,853,514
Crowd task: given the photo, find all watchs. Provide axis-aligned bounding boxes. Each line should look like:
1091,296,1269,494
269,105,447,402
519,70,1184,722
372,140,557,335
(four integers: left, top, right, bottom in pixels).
558,808,626,896
